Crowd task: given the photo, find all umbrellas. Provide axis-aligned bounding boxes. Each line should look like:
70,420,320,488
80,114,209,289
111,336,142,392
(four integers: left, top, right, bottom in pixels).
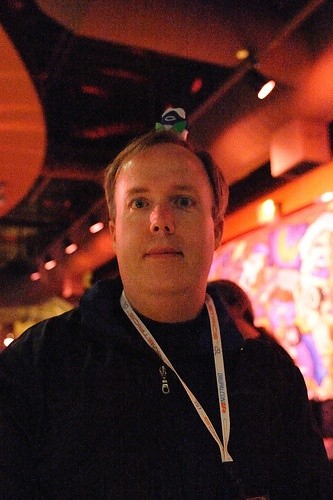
0,27,45,218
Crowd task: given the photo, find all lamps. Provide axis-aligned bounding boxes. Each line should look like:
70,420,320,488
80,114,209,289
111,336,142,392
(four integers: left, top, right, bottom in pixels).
30,266,40,281
43,254,57,271
63,237,79,254
87,214,104,235
242,68,275,100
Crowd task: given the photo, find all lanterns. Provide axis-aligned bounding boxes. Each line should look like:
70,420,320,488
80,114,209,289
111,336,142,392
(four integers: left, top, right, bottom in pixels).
269,119,328,178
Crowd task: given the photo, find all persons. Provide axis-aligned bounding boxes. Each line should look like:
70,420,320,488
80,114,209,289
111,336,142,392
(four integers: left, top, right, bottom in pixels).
207,278,295,366
0,125,332,500
